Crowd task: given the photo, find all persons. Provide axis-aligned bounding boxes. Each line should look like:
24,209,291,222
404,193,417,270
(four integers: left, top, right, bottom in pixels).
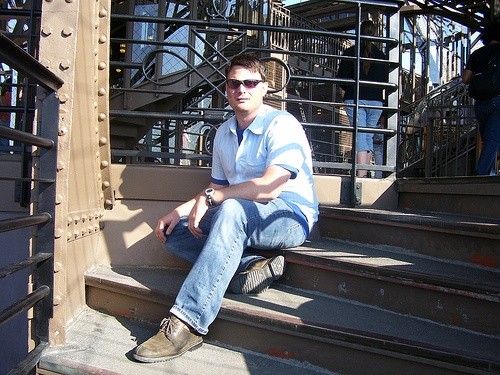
132,52,322,365
335,17,390,177
462,16,500,176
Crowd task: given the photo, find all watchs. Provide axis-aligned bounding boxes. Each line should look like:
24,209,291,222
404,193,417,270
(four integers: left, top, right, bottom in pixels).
204,187,217,208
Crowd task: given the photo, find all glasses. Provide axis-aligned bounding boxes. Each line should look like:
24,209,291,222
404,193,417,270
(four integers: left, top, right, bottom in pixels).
224,79,265,89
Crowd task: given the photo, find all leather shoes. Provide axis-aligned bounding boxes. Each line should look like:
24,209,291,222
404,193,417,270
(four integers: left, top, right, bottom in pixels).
132,314,204,364
229,255,286,295
